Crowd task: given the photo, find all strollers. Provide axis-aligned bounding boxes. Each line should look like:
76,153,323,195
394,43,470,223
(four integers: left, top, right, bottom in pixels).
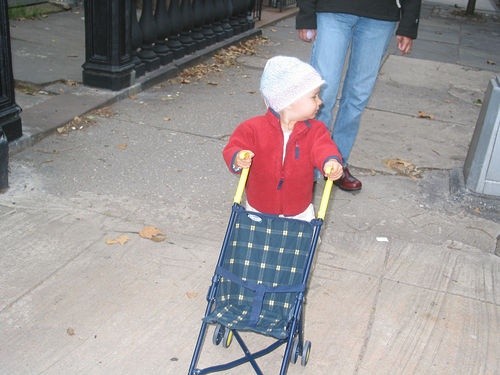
187,151,334,375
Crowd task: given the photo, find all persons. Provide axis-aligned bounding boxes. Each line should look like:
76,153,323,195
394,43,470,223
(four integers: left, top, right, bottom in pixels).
223,56,345,248
296,0,421,191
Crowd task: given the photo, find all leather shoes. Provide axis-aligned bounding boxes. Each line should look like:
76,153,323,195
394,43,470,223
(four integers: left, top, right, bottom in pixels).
323,166,362,192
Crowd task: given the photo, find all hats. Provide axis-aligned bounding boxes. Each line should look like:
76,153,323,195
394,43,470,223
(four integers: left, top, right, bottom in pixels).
260,56,325,113
